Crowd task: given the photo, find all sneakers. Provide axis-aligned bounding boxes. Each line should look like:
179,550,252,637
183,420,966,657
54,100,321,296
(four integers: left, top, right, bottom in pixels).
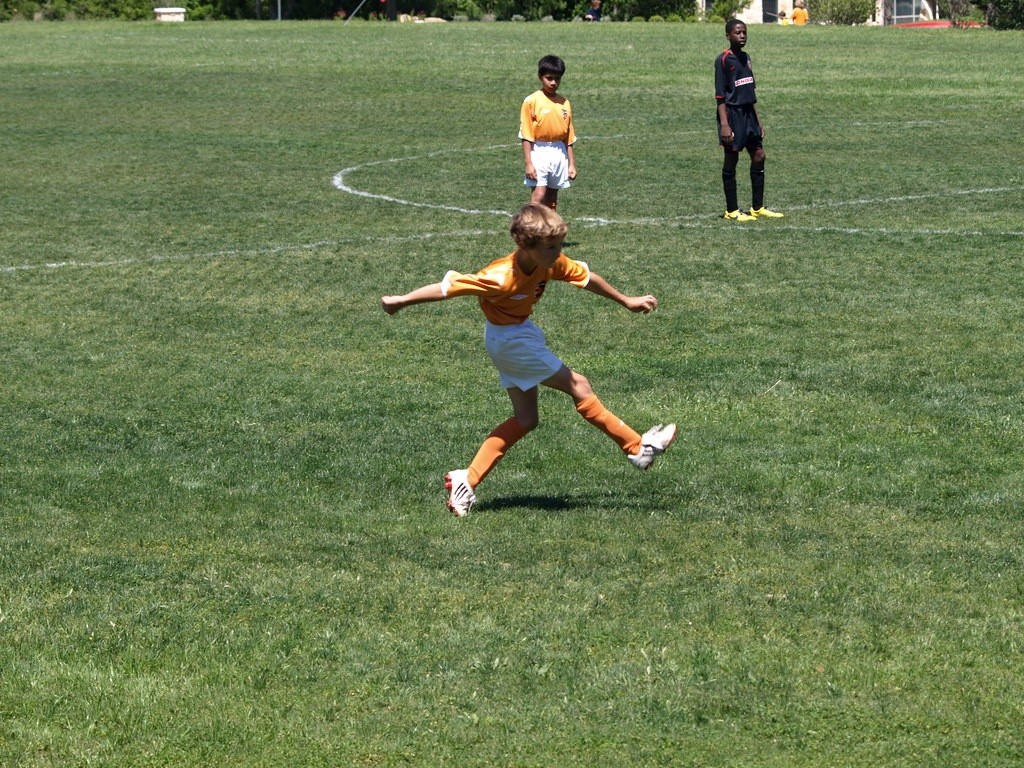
628,423,677,470
724,211,757,222
749,206,784,218
444,469,475,518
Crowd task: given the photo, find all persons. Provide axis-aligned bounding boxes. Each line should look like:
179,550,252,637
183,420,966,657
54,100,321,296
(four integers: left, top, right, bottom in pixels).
518,56,577,210
779,11,789,26
713,19,785,222
791,0,810,25
585,0,602,22
380,202,677,518
